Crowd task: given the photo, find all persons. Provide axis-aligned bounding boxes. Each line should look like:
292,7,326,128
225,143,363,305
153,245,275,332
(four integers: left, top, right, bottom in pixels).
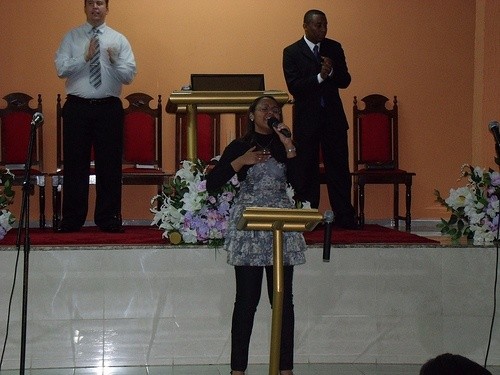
282,10,357,229
206,95,310,375
54,0,137,234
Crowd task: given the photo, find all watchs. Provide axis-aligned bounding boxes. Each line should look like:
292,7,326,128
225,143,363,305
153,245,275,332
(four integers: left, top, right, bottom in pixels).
287,146,296,152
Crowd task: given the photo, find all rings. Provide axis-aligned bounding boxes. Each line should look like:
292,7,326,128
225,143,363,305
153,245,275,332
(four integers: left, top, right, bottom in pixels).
259,158,260,160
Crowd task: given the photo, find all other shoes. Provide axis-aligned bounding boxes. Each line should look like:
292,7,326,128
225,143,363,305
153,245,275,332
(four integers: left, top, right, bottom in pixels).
111,225,124,232
56,226,74,232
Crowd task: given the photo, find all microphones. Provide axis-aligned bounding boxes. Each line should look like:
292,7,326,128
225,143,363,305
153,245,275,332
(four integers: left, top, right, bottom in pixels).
323,210,334,262
31,113,44,125
488,120,500,148
267,117,291,138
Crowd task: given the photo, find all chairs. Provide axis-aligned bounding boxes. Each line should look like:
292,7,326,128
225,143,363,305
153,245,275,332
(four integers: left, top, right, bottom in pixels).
0,92,416,236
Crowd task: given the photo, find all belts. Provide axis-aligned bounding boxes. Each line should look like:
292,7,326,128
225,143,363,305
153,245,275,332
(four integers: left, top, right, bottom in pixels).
68,94,116,105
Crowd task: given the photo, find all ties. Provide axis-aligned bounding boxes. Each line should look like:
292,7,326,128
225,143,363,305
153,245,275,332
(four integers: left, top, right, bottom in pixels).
313,45,319,57
89,27,101,89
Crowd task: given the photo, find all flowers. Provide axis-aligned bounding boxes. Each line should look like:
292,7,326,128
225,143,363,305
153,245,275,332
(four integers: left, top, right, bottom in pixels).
0,168,19,236
148,156,312,258
432,164,500,246
